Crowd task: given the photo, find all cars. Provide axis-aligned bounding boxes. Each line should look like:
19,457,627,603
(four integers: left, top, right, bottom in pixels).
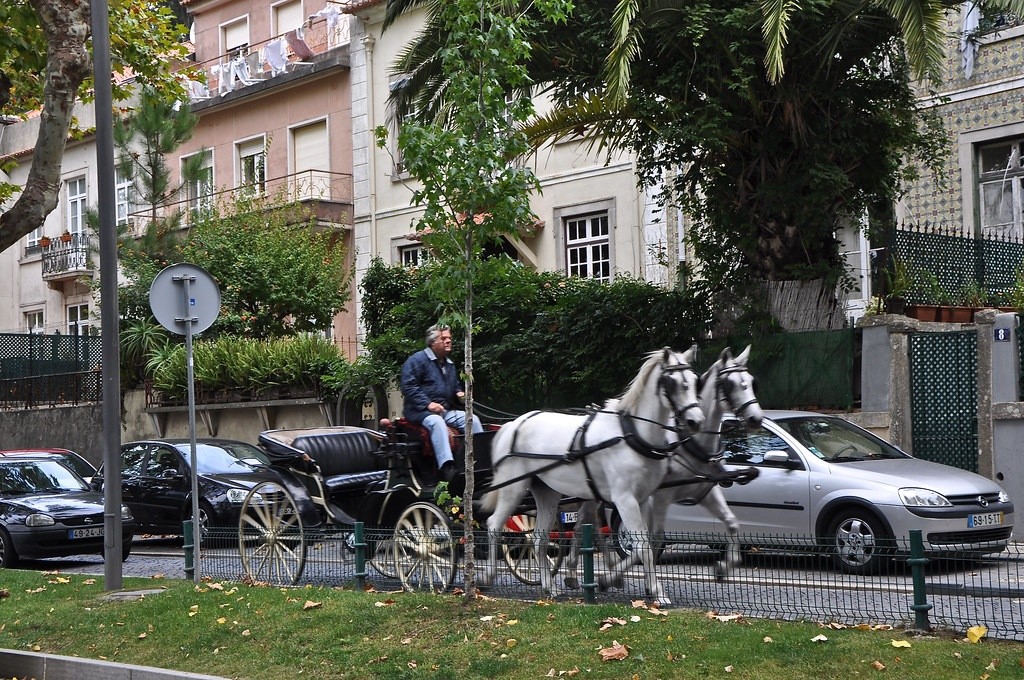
0,448,136,569
90,438,323,547
342,424,612,553
577,408,1014,573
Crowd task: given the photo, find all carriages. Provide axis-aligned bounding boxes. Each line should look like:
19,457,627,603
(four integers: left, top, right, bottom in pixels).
237,344,764,609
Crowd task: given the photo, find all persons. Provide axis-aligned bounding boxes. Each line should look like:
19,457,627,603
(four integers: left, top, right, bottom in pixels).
400,324,484,469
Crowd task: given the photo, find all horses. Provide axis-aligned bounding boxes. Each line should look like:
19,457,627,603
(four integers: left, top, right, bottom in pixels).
481,344,705,607
565,345,763,590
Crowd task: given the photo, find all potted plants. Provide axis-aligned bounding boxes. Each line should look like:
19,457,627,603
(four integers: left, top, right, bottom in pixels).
37,236,51,247
60,229,71,242
938,287,953,322
882,252,916,314
972,286,993,322
987,292,1019,312
911,269,942,322
951,275,979,323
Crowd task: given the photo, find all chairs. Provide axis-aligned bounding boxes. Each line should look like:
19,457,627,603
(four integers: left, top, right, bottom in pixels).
159,453,179,473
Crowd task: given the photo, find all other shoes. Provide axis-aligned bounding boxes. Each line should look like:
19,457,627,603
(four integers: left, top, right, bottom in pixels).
442,461,460,483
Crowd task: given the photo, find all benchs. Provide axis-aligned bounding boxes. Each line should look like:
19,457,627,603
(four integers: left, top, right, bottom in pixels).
393,418,462,458
291,432,386,496
